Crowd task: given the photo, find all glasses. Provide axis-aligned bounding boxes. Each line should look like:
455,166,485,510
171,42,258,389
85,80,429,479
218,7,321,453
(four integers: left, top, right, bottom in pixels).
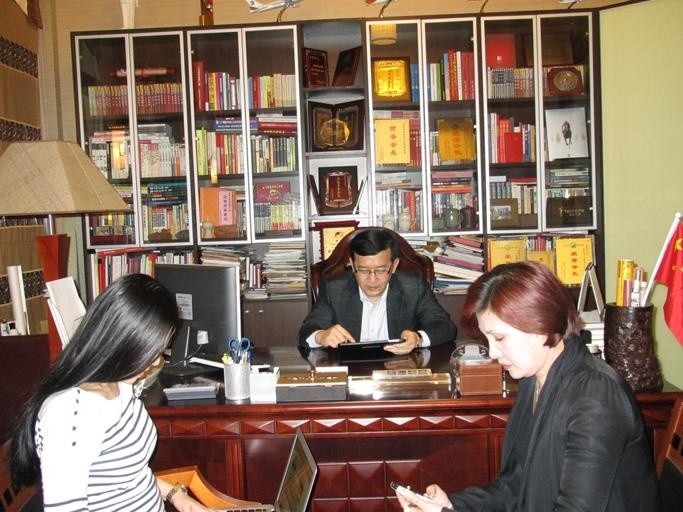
353,261,393,275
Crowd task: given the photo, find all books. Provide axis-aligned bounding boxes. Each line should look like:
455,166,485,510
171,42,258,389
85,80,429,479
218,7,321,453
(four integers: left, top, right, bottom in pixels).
43,276,87,352
86,61,308,296
306,98,365,152
318,166,359,215
302,46,362,88
372,49,594,297
308,220,357,263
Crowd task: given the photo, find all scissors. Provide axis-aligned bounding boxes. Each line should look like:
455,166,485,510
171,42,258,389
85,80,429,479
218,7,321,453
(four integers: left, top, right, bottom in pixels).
228,337,251,364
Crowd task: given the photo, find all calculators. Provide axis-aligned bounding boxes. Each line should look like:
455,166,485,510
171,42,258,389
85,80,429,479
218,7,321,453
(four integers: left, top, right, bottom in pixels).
162,381,221,401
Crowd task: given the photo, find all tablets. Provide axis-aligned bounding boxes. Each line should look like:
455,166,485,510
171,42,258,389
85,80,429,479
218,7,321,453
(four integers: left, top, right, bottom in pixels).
338,338,406,349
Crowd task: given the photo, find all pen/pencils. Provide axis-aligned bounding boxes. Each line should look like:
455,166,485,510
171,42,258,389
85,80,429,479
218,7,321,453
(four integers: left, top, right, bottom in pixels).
221,341,255,364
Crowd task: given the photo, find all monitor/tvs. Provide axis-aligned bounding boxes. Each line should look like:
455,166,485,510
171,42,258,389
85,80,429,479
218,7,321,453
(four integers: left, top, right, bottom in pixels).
153,262,243,377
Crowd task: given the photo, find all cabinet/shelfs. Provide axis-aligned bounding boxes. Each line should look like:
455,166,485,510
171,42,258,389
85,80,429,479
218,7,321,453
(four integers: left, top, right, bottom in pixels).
67,22,310,350
358,6,603,294
297,19,370,260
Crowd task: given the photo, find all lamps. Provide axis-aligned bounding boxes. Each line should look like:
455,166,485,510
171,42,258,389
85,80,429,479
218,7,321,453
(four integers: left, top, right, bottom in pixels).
1,140,136,361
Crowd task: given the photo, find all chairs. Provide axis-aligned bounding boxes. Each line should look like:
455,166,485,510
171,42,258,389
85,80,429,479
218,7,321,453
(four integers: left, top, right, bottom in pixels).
306,226,437,307
654,393,683,512
1,438,262,512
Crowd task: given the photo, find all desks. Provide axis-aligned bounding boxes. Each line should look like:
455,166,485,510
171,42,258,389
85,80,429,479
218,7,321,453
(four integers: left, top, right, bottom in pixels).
139,358,682,512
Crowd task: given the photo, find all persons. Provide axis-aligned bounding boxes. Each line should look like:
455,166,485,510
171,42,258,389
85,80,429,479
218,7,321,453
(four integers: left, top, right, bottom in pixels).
10,273,218,512
396,261,659,511
298,229,457,355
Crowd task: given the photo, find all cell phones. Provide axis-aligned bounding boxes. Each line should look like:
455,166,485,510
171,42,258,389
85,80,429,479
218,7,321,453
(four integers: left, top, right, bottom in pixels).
390,480,441,505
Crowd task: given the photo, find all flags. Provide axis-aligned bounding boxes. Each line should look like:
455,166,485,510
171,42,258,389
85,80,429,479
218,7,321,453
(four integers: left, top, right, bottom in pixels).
653,211,683,349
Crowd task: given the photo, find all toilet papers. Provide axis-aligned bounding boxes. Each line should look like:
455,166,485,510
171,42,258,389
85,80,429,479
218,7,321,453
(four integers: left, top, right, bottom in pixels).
5,265,32,336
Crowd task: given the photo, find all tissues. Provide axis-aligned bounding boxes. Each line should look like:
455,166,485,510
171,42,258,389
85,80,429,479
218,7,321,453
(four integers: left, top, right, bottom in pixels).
249,364,280,404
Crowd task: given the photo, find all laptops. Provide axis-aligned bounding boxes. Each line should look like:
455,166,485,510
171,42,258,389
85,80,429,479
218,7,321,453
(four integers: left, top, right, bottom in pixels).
208,426,318,512
